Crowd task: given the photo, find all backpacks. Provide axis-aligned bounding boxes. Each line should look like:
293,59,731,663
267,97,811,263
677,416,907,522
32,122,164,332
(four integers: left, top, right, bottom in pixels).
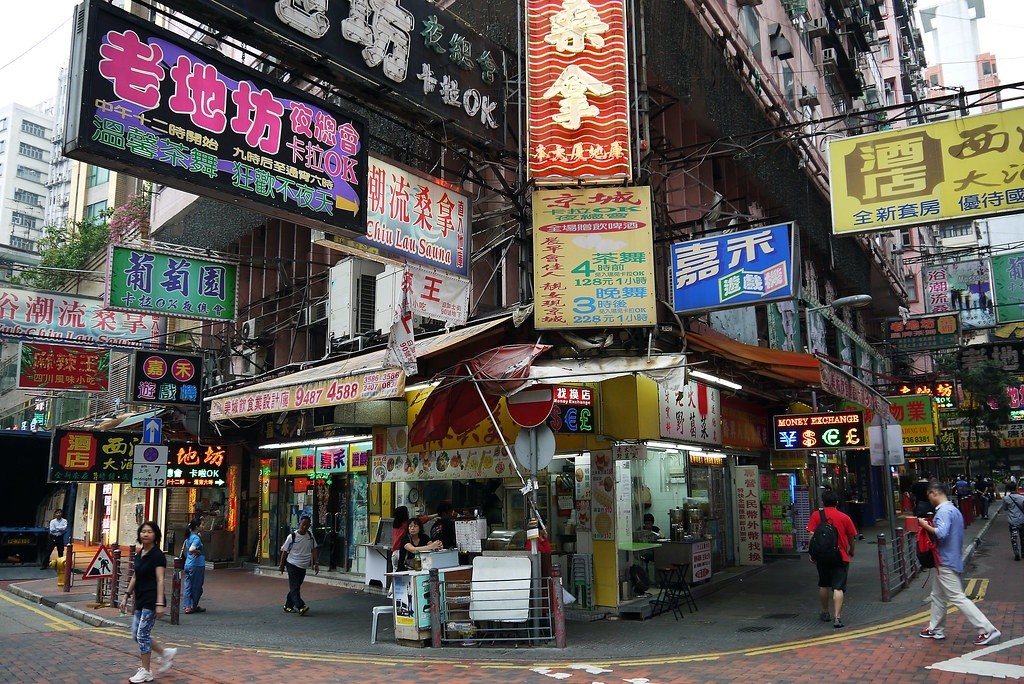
916,519,943,569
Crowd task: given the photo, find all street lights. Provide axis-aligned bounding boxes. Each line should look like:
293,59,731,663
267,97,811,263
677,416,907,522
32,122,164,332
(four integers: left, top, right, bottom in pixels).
803,294,875,502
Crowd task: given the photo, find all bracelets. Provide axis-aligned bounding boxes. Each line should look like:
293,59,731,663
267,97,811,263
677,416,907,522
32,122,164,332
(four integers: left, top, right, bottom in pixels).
155,602,165,607
125,591,131,598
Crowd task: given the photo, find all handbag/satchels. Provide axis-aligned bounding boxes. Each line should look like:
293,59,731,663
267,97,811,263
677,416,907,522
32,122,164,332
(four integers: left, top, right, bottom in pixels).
809,507,838,566
562,586,576,605
174,539,188,569
916,501,934,517
403,558,415,569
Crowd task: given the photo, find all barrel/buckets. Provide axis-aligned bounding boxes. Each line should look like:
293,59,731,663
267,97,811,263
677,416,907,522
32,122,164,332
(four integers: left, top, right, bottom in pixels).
668,506,703,540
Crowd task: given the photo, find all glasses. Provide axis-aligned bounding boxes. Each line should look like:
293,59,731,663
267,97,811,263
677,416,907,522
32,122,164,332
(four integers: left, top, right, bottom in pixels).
645,522,652,525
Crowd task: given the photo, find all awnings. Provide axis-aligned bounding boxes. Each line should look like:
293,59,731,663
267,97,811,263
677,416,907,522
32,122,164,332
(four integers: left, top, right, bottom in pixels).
203,314,511,423
683,330,901,572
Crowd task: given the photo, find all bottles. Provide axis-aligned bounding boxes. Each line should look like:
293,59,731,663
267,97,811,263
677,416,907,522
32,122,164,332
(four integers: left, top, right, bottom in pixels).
691,503,708,515
414,551,422,571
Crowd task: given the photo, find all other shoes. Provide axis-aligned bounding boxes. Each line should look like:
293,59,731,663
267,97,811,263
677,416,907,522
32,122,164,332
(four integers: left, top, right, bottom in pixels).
185,607,192,613
284,607,297,613
1015,552,1021,562
40,566,48,570
387,593,394,599
299,606,309,616
191,606,206,612
984,515,989,520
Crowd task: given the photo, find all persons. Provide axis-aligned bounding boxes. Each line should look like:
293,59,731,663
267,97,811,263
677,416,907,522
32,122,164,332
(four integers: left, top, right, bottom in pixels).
40,508,68,570
636,513,664,539
385,506,443,599
279,516,320,614
525,520,552,555
917,481,1002,646
954,474,995,520
1002,481,1024,561
182,517,208,614
118,521,178,683
805,489,858,627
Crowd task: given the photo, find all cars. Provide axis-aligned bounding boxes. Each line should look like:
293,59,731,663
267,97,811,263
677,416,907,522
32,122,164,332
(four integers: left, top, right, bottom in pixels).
1011,476,1024,496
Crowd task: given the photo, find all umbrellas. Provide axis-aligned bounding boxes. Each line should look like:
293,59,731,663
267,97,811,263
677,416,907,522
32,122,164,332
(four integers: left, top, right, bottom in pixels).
407,343,553,535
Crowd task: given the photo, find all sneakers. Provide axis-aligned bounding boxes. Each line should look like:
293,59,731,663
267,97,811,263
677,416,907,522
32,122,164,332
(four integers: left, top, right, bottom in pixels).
919,627,946,639
819,610,831,622
129,666,155,684
973,629,1002,645
158,647,176,672
833,616,844,627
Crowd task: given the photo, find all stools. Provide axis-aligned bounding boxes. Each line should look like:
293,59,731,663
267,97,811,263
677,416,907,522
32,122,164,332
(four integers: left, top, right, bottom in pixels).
370,606,394,646
670,562,698,613
652,567,684,621
569,554,592,612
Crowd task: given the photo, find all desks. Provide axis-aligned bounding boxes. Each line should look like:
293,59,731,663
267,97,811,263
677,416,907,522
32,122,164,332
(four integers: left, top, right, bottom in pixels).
617,543,662,600
470,557,531,648
383,566,472,648
654,537,711,583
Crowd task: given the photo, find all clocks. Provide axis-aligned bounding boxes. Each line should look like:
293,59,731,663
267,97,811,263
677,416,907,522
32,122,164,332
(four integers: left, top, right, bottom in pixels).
408,488,419,504
